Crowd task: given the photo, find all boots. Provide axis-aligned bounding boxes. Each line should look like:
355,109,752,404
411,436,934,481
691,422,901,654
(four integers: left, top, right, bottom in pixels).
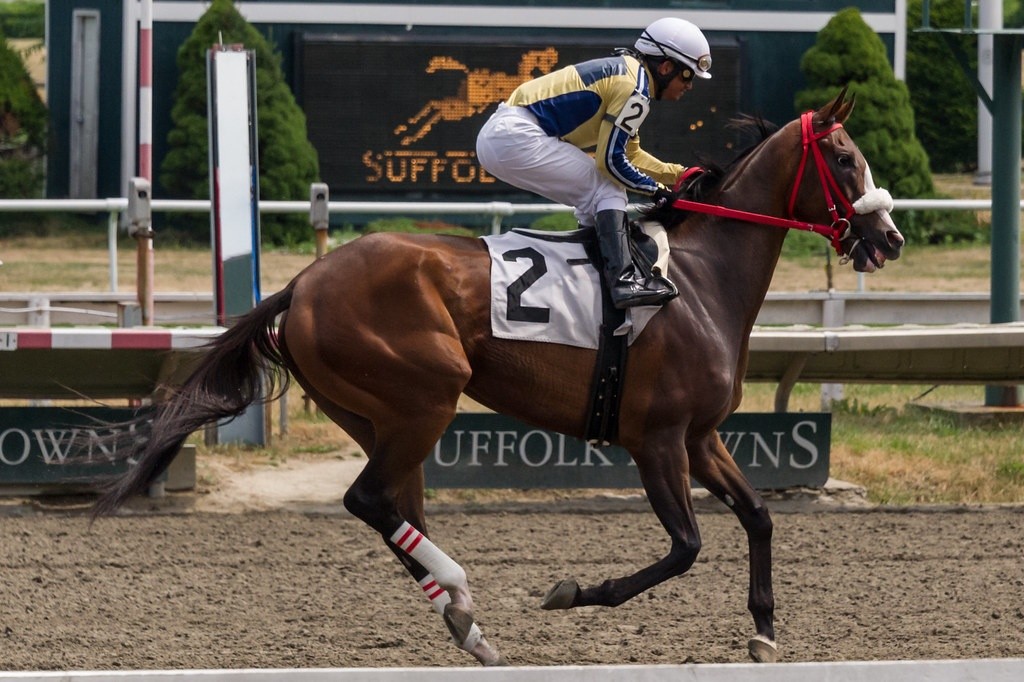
594,209,681,309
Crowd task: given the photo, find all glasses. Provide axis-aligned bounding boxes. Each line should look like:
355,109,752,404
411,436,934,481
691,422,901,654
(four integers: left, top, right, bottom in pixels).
661,57,696,83
697,54,711,72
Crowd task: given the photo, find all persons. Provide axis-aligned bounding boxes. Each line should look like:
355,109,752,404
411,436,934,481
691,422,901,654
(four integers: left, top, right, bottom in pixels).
476,18,712,310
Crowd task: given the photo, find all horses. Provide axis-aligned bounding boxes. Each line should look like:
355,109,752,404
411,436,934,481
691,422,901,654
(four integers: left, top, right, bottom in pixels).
28,85,904,665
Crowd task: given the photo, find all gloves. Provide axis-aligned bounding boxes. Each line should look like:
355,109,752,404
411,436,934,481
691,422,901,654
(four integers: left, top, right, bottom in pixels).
650,182,677,209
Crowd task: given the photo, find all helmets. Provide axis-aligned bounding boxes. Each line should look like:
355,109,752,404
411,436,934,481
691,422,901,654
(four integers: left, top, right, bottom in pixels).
634,17,712,79
